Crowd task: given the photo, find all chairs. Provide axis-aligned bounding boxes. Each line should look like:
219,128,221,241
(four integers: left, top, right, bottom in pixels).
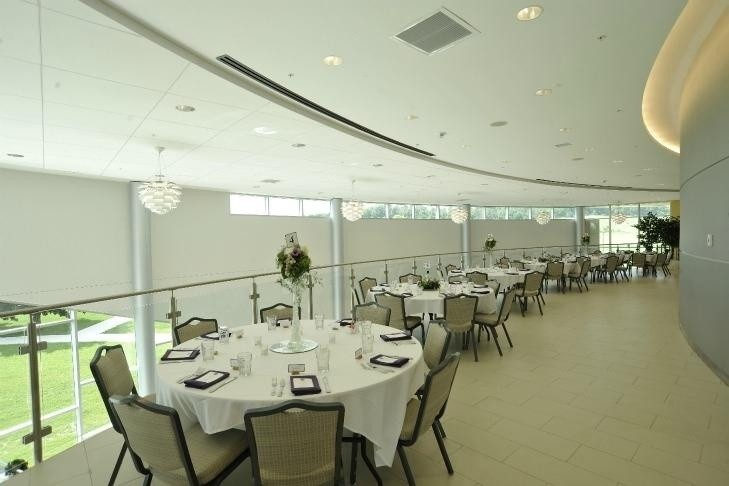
243,394,352,486
442,291,480,363
104,392,252,486
352,247,490,288
260,302,304,323
374,289,426,346
385,353,468,486
89,342,160,485
492,248,677,319
465,286,518,356
173,314,220,345
415,319,452,438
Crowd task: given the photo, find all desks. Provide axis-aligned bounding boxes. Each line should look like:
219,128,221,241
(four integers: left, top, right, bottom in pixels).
447,267,540,293
366,282,496,318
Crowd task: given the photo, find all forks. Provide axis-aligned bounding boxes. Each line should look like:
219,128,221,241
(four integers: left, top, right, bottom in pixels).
271,375,277,396
360,362,394,375
176,366,207,384
278,377,287,397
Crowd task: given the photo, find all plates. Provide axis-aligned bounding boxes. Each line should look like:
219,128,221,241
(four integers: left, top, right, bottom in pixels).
506,272,520,276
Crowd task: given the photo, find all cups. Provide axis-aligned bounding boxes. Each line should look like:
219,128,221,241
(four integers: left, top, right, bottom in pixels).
316,343,329,374
328,330,336,345
266,316,277,333
350,319,360,335
314,312,325,330
438,281,474,295
228,352,253,377
450,269,461,272
463,263,498,273
360,319,374,355
288,318,304,354
385,279,424,297
201,340,216,361
253,335,262,346
260,344,269,356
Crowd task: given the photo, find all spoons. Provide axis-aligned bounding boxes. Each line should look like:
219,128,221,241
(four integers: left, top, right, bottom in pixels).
389,340,417,346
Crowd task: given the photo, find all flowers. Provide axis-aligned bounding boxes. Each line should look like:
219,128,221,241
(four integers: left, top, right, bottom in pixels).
482,232,497,249
274,241,322,320
582,232,591,244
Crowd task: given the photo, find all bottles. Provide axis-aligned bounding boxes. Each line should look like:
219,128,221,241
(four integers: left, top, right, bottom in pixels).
216,319,230,345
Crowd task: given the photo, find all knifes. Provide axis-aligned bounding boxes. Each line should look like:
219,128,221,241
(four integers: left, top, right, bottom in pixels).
208,377,238,394
323,377,331,393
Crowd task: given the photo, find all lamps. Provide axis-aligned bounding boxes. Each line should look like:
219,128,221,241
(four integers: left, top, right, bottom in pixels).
534,198,552,227
447,192,471,224
339,176,368,223
136,146,185,216
610,201,627,226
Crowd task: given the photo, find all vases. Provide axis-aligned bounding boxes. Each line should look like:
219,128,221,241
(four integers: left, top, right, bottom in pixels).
289,283,305,347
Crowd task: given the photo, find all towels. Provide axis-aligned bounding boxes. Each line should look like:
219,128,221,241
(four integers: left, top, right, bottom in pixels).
200,331,233,340
289,373,322,396
337,318,355,326
160,348,200,361
275,317,292,328
368,353,410,367
379,331,412,342
182,369,231,389
